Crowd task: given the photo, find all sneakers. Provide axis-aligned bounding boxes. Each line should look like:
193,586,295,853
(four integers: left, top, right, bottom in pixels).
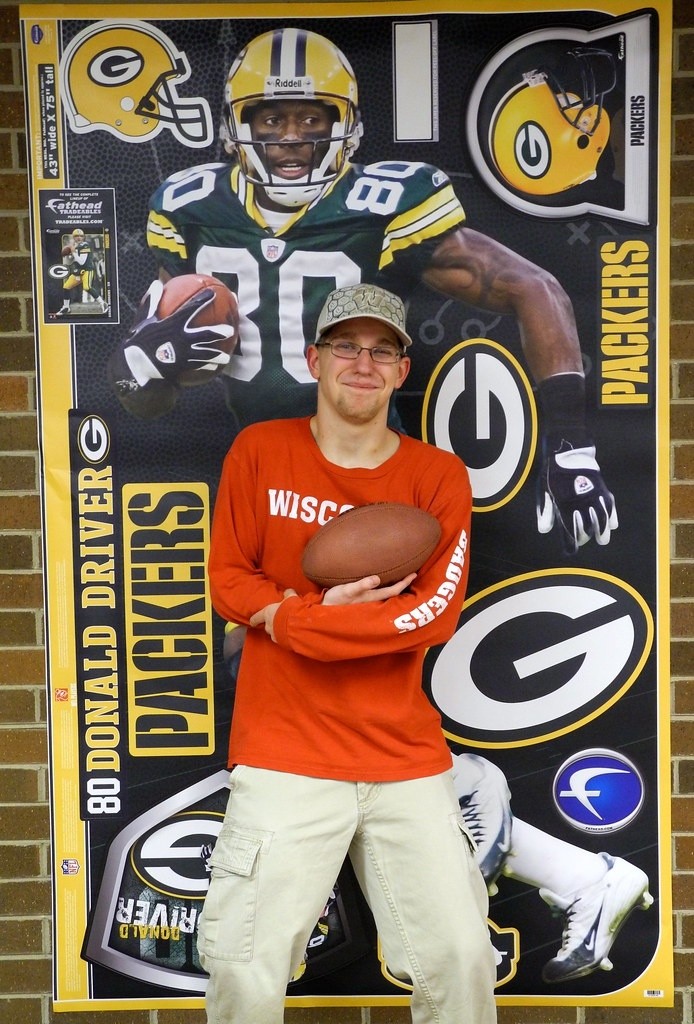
452,753,515,897
539,852,654,985
55,307,71,316
101,302,109,313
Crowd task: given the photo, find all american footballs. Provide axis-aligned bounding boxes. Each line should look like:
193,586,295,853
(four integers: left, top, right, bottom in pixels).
155,273,240,386
300,503,442,589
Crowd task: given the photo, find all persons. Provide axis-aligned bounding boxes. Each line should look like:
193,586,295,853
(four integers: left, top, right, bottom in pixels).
62,25,657,983
197,284,497,1024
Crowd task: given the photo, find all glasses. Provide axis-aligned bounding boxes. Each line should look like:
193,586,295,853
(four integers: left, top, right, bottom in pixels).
74,235,84,237
317,341,404,365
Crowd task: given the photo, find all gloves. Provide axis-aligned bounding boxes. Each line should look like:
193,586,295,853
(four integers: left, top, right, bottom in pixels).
105,279,234,398
533,371,619,553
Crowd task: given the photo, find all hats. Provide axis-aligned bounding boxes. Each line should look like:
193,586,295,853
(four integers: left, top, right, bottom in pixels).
314,282,413,347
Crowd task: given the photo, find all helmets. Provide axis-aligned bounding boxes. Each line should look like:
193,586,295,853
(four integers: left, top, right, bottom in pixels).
218,26,365,208
72,228,85,237
